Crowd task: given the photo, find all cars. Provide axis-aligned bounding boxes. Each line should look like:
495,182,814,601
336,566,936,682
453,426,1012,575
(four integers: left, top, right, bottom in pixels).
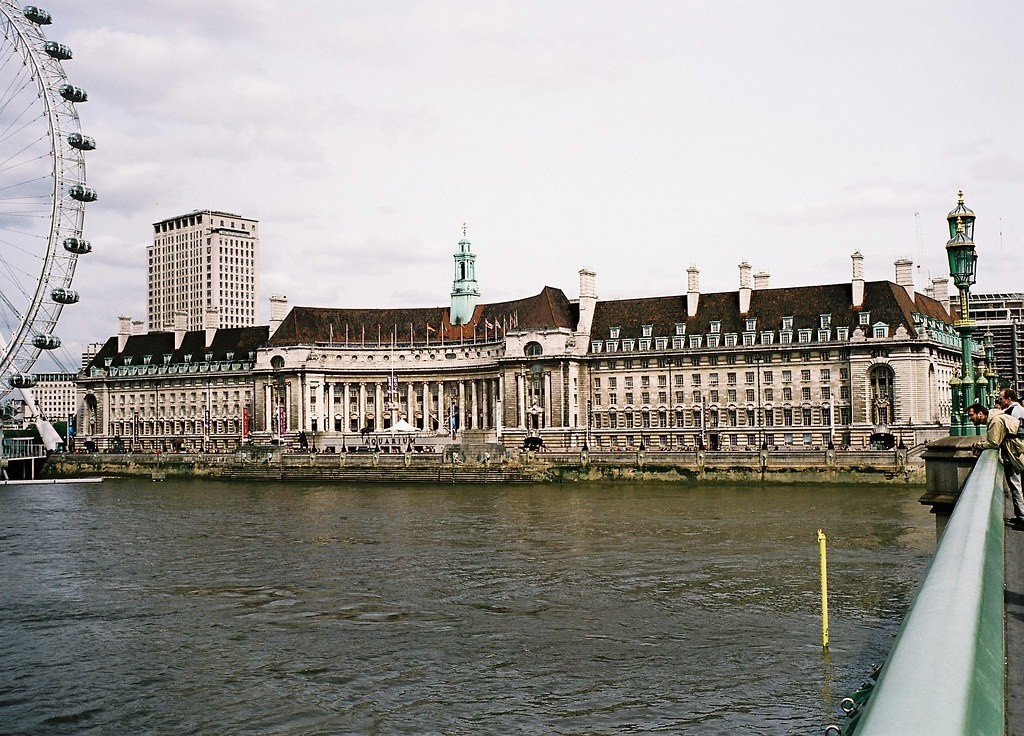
174,447,191,453
104,445,127,453
518,437,547,454
411,446,432,453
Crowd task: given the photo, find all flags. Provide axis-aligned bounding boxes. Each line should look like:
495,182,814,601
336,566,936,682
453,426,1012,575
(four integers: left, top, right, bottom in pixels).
495,318,502,329
427,323,438,333
411,325,417,336
485,320,494,330
442,323,452,335
461,323,468,332
503,311,518,334
474,322,482,333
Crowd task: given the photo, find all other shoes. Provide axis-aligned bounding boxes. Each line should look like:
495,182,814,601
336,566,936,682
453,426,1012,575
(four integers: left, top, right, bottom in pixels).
1010,518,1024,523
1012,523,1024,531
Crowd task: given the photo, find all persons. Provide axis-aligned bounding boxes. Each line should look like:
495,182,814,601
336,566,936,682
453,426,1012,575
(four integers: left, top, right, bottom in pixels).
73,443,894,463
965,388,1024,530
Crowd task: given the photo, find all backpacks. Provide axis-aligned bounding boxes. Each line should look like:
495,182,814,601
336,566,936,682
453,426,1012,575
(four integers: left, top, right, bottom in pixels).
1017,417,1024,438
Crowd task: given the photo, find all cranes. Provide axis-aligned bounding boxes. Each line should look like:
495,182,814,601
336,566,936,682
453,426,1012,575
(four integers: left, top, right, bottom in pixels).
914,211,927,296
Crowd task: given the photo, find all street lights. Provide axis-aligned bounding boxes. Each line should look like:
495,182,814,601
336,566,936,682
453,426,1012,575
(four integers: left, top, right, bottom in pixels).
945,190,998,437
312,430,317,452
